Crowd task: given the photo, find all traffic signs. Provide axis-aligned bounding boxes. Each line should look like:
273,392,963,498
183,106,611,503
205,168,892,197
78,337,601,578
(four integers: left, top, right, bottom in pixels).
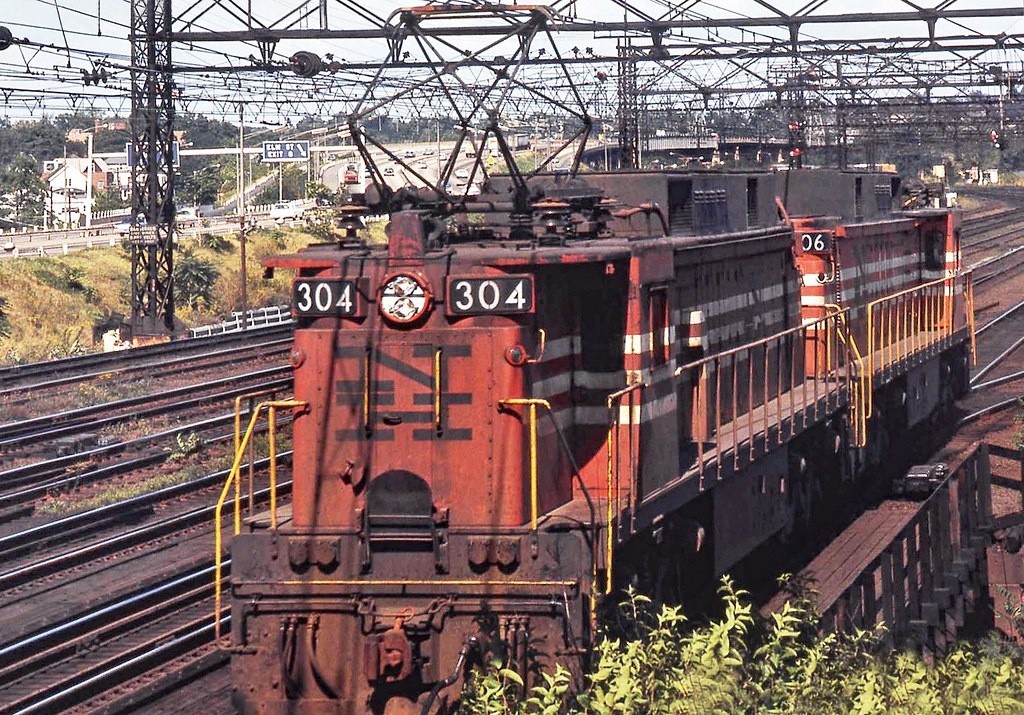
260,141,311,162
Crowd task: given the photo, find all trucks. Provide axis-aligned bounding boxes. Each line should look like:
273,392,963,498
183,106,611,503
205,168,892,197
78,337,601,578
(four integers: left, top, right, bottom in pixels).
507,133,532,152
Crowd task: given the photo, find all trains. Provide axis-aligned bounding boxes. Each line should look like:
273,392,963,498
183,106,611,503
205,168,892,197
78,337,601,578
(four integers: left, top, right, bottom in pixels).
215,165,972,715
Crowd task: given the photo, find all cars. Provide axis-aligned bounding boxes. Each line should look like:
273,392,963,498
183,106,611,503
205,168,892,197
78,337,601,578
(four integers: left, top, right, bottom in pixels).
177,210,197,219
269,203,303,221
112,216,147,238
344,137,502,185
